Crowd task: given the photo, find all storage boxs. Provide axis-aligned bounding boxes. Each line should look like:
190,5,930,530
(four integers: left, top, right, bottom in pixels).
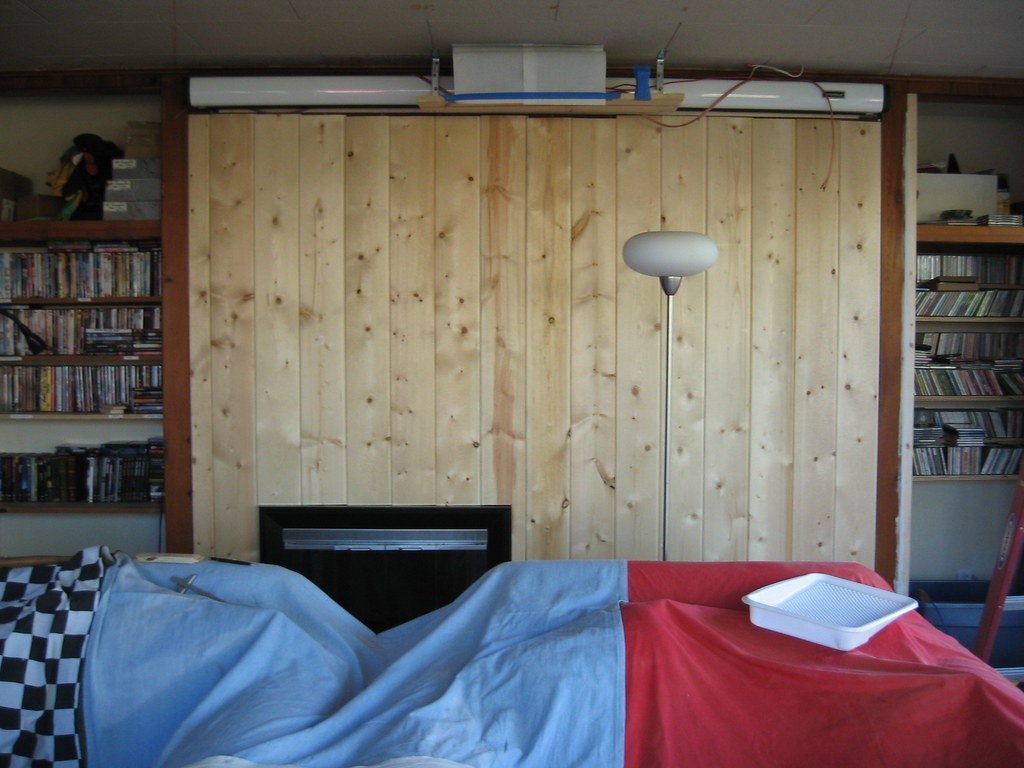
909,581,1024,667
0,168,66,219
101,121,160,217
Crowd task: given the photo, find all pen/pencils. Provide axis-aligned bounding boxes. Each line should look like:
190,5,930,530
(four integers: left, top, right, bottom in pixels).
208,556,253,565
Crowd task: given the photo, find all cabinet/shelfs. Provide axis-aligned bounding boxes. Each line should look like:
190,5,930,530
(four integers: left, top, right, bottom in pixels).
0,220,173,516
913,222,1024,482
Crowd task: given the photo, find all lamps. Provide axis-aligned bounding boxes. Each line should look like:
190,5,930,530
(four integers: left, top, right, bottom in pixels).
623,231,719,560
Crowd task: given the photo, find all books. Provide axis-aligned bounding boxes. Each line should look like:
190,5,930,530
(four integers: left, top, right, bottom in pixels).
0,242,165,504
909,213,1024,476
